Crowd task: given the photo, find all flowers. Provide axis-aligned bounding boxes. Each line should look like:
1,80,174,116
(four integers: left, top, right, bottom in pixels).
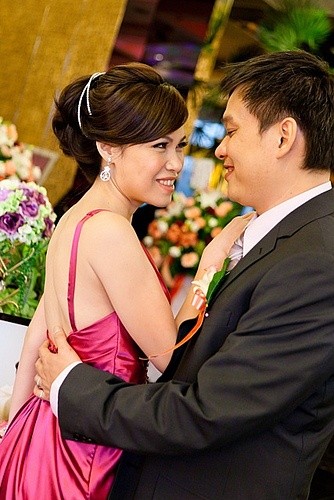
191,257,231,313
144,189,242,287
0,181,57,315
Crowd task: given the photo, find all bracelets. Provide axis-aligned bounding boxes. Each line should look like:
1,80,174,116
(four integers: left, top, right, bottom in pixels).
190,280,201,287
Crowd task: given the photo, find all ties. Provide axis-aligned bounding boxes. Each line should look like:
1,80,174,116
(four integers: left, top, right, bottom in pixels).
226,228,245,271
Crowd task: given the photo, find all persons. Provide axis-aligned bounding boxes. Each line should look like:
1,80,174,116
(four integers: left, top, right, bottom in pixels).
34,48,334,500
0,62,258,500
